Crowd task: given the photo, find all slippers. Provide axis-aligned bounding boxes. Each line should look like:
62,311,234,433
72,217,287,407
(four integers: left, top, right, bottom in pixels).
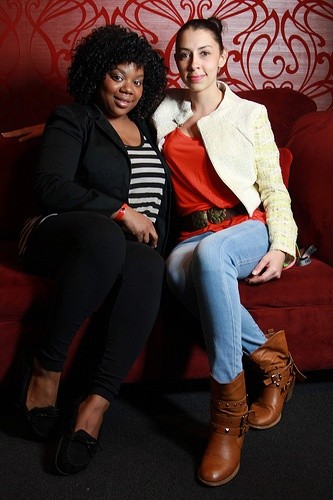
252,328,295,427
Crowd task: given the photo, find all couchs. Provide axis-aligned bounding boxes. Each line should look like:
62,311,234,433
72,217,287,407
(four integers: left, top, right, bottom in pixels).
0,89,332,395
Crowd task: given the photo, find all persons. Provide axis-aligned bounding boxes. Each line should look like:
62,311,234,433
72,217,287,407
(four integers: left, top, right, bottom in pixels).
151,14,307,487
1,22,172,476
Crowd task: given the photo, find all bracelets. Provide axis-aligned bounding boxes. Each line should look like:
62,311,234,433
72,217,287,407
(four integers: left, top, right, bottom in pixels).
115,203,128,222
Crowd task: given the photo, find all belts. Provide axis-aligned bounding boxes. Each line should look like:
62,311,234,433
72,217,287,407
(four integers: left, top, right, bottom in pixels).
178,202,248,232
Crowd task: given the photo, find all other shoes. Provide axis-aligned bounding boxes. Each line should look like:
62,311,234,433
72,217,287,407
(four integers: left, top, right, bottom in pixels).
14,369,55,430
53,429,95,474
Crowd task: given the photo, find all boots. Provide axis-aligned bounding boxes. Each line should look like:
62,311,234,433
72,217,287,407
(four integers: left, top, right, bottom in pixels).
198,373,249,487
